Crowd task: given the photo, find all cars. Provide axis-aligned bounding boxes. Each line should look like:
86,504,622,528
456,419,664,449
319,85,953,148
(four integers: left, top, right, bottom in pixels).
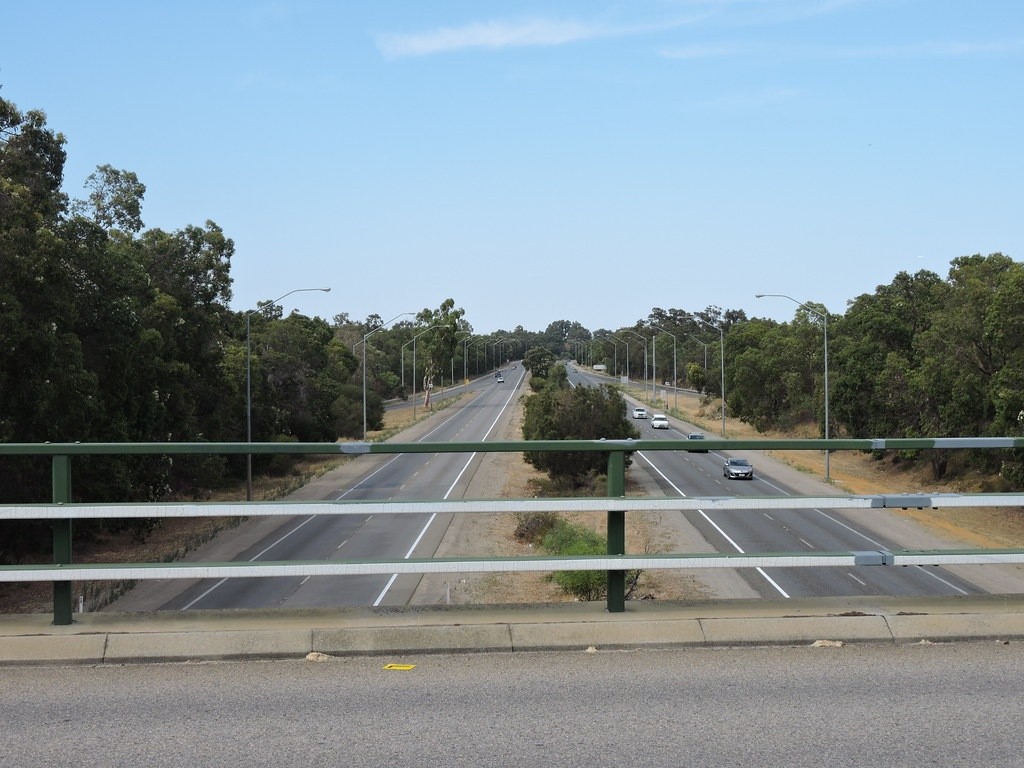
724,458,753,480
632,406,648,418
497,377,505,383
650,413,671,428
492,372,501,378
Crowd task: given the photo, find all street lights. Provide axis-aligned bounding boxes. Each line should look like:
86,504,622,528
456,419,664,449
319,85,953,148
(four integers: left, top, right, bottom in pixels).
619,330,648,405
754,294,830,481
683,333,707,372
605,333,629,391
676,315,725,438
245,288,337,494
596,334,617,378
642,325,680,409
363,312,417,443
413,324,452,417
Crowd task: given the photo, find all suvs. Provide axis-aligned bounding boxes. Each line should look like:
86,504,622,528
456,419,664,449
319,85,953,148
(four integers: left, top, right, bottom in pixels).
685,431,709,453
